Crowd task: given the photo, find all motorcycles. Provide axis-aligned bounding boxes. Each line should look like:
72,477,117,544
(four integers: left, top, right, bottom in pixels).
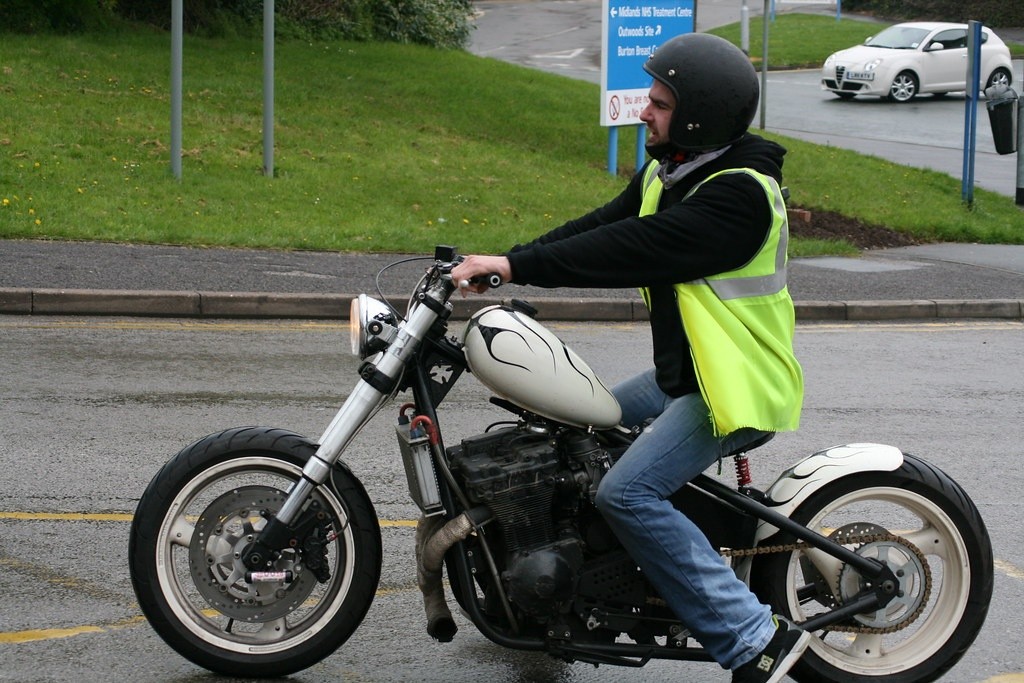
128,244,995,683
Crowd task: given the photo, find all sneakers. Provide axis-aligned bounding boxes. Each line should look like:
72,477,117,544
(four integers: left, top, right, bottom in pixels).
731,614,814,683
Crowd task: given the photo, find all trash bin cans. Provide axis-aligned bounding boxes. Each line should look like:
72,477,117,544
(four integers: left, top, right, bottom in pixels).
986,88,1017,155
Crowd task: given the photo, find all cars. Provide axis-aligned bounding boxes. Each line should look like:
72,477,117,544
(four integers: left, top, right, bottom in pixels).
819,20,1016,105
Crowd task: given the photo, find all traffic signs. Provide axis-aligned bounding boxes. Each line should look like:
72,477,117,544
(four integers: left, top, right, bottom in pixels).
600,0,698,126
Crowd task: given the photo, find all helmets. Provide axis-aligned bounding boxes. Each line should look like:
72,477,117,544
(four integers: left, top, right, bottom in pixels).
643,33,759,151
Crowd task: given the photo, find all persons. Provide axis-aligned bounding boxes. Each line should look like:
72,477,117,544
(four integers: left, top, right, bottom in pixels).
449,34,810,683
